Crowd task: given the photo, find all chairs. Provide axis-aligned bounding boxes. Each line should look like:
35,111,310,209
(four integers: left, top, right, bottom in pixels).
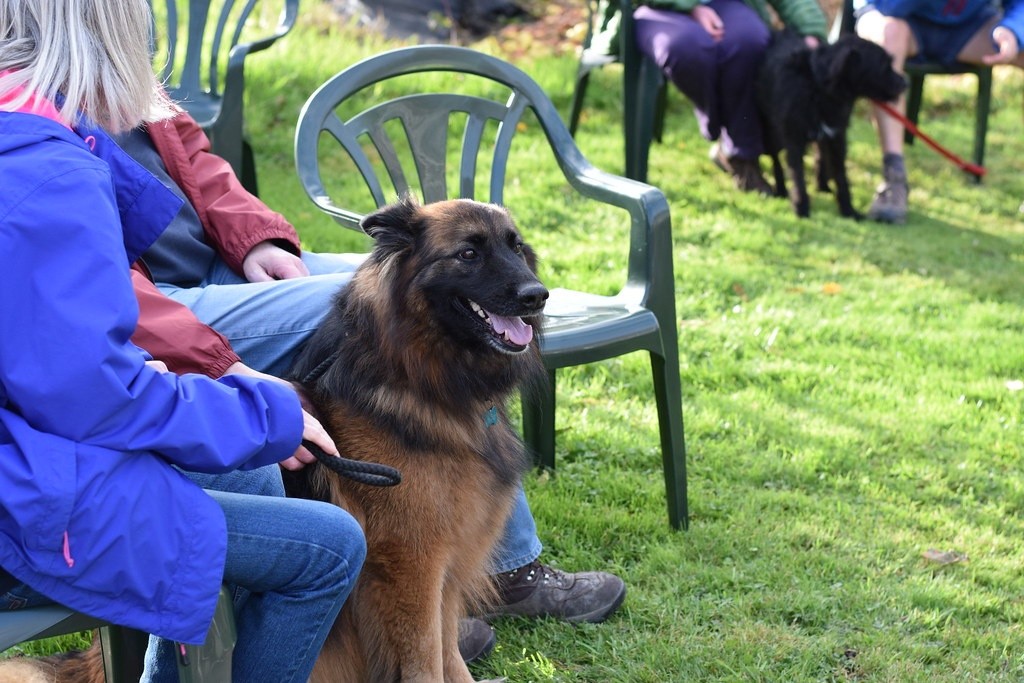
826,0,996,182
566,2,700,195
288,42,692,532
138,1,302,188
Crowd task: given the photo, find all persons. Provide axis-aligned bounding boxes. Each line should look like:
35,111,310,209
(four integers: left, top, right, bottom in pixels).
51,75,629,665
0,0,367,682
848,0,1024,226
589,0,830,197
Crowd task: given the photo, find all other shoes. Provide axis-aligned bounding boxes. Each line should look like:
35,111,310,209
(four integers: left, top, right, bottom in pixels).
708,143,774,195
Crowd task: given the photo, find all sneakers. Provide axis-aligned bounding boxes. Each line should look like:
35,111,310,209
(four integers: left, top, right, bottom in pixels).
458,560,625,664
868,181,910,225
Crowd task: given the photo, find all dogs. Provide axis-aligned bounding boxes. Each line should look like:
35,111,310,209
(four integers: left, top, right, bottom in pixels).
752,30,907,222
0,194,550,683
